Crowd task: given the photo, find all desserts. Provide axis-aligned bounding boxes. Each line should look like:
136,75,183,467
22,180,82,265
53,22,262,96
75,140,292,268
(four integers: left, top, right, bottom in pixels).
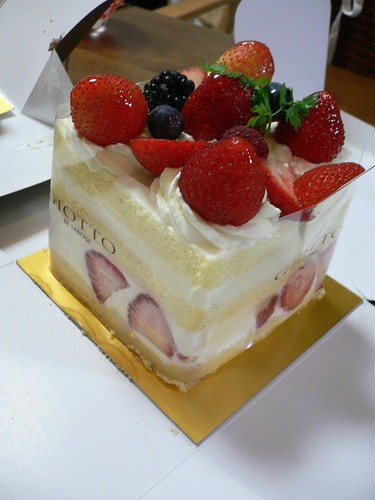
46,41,375,395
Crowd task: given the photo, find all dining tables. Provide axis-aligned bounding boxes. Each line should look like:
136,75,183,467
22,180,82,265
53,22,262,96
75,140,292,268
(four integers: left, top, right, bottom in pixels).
0,4,375,500
